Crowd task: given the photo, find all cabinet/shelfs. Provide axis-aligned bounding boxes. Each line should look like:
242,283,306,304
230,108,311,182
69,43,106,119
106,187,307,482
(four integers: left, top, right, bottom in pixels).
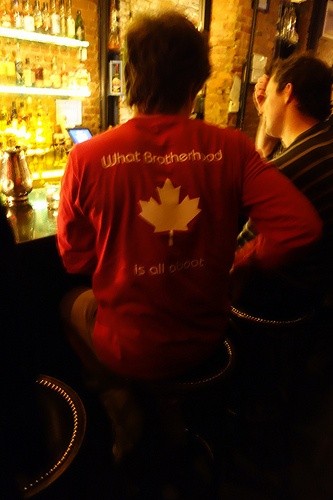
0,26,91,180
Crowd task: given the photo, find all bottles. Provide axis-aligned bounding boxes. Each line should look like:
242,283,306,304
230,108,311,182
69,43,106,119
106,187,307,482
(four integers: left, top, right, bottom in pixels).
111,62,124,97
0,96,70,148
1,38,95,90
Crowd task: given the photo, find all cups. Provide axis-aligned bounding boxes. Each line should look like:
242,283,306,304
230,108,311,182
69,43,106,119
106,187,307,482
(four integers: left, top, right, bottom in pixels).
46,181,63,210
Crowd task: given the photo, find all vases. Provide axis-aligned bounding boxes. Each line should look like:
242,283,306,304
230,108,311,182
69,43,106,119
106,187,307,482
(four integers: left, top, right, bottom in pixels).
2,145,34,202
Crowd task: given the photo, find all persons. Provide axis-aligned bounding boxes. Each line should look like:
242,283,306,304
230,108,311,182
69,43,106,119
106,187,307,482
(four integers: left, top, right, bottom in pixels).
226,54,333,311
56,10,323,460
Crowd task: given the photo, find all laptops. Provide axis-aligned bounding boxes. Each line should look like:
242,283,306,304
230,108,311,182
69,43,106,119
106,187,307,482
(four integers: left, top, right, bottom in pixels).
67,128,93,144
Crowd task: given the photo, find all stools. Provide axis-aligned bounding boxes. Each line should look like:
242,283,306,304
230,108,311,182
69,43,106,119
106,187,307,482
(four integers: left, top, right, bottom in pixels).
226,302,321,492
133,337,233,500
20,374,90,500
